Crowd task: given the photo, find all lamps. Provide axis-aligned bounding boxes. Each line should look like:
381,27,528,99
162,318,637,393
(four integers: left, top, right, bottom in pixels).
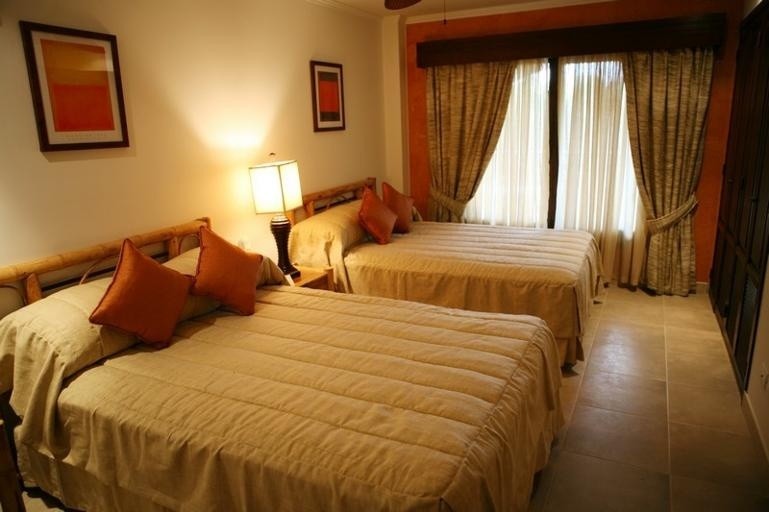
248,152,305,279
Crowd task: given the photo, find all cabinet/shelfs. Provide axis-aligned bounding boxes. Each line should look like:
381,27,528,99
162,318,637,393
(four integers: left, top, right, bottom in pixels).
707,1,769,398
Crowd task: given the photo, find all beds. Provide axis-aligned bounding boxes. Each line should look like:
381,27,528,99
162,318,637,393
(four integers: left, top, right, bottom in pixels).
0,216,565,512
287,176,611,374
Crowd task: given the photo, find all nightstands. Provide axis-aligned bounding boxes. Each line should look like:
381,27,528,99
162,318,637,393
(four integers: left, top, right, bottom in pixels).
292,266,329,290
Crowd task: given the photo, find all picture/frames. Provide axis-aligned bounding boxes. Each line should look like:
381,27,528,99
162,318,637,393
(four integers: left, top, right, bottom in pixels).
18,20,130,152
310,60,345,133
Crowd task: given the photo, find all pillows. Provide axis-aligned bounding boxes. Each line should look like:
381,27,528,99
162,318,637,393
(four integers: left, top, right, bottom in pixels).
381,182,417,234
357,183,398,245
0,245,203,460
286,196,367,294
88,238,197,350
190,225,263,317
